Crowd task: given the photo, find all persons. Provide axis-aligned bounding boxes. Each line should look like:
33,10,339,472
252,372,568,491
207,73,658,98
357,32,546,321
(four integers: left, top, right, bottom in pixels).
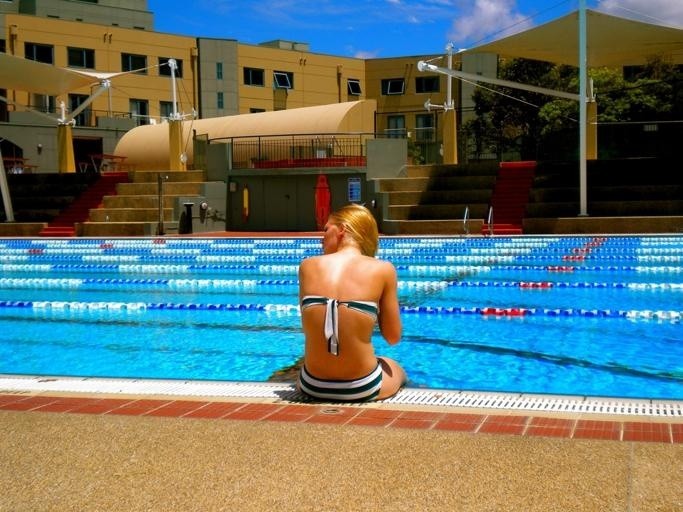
296,203,409,405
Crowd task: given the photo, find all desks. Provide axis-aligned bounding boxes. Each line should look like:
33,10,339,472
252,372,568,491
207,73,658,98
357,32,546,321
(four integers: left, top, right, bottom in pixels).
2,157,30,174
89,154,128,173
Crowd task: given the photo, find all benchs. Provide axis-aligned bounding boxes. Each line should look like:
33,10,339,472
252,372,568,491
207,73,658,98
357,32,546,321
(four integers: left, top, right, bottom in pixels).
79,162,88,173
6,163,39,173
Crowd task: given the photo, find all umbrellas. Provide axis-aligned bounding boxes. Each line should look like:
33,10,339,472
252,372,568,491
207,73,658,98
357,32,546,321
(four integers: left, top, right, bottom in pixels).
416,6,683,216
0,50,198,222
